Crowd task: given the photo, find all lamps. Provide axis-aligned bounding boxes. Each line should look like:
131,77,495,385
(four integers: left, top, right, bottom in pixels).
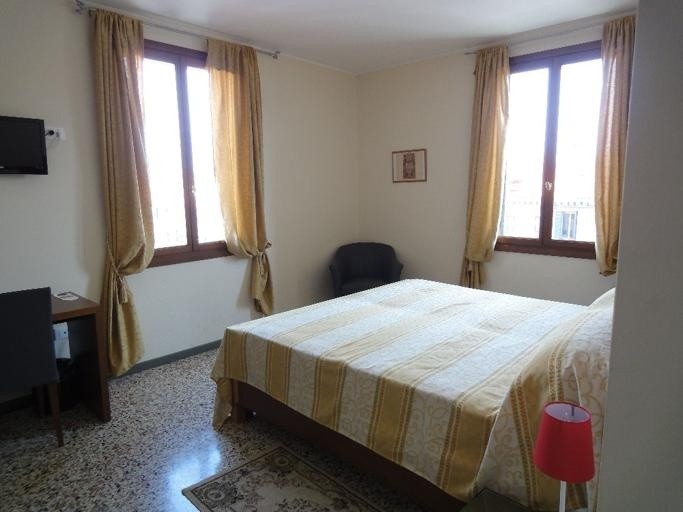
532,402,595,512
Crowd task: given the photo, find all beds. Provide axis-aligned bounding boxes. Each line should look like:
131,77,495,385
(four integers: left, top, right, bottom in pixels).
213,277,616,512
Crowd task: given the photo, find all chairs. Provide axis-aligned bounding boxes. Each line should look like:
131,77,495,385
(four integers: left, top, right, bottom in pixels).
330,242,403,297
0,286,64,446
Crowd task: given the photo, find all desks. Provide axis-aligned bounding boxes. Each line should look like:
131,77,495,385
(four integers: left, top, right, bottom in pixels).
50,292,112,424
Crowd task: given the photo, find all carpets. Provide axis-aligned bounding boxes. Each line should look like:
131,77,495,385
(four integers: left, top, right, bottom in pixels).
181,444,384,512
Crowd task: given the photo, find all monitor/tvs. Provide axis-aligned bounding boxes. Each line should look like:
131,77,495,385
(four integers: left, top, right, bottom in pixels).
0,116,48,175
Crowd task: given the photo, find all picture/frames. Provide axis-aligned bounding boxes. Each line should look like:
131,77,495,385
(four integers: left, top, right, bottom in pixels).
391,149,427,182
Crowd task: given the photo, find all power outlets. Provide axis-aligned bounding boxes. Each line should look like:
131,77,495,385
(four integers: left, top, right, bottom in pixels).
45,127,65,139
